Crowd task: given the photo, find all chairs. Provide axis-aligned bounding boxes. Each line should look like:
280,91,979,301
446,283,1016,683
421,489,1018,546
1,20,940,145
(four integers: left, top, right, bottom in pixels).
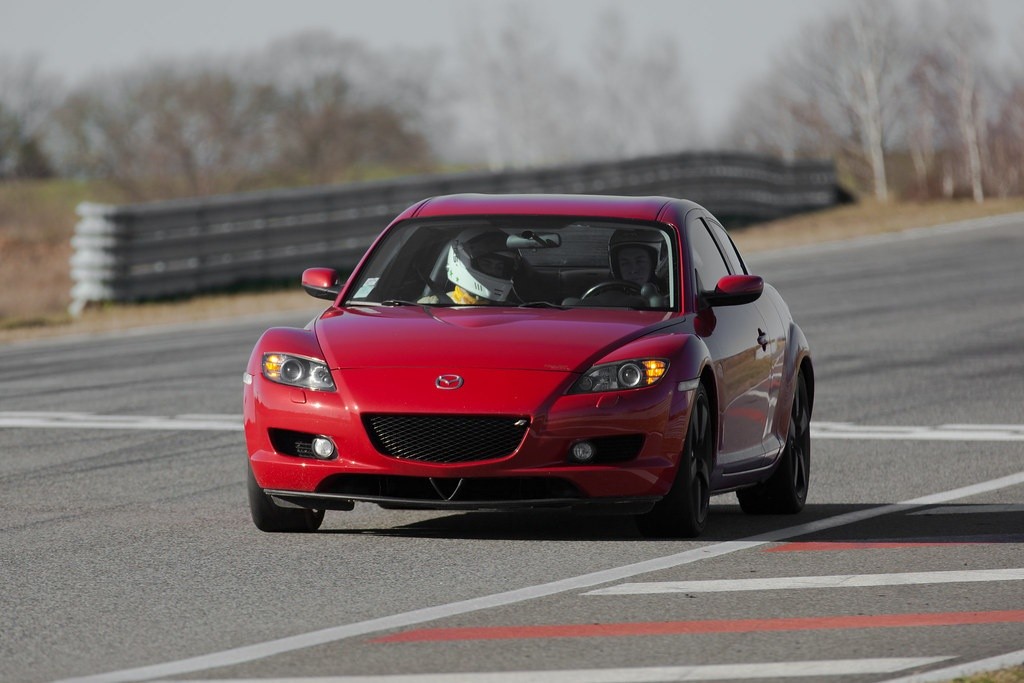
422,238,543,301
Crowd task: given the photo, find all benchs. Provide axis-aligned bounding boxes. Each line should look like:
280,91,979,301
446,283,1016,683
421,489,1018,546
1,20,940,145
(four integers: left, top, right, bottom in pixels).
533,266,612,306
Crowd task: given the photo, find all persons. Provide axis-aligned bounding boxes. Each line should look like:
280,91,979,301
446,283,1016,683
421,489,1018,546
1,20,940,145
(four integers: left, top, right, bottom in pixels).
607,227,677,302
412,223,522,307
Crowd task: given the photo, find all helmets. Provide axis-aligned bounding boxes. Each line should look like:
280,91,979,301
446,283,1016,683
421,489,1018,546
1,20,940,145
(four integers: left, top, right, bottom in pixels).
607,227,669,281
445,225,522,301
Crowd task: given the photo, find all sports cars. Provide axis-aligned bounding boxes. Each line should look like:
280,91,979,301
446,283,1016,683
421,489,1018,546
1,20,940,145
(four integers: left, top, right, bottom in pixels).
242,194,815,539
